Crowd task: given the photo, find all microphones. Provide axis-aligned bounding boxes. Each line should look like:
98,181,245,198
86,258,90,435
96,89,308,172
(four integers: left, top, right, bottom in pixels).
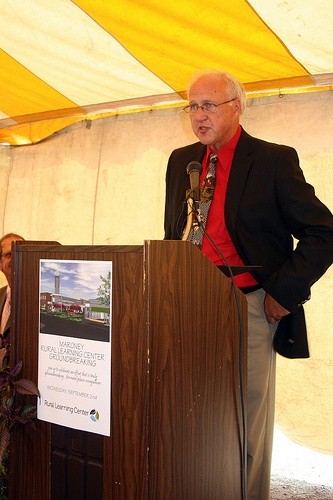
187,161,203,224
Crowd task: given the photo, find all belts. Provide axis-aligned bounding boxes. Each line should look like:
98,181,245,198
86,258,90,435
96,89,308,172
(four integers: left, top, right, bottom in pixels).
240,284,263,294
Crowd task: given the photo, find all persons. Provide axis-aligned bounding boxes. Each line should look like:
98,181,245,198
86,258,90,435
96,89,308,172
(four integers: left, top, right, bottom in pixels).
163,72,332,499
0,232,24,499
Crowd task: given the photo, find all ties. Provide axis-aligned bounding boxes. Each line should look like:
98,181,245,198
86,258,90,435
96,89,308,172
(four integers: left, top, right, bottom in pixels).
0,290,12,335
186,152,218,250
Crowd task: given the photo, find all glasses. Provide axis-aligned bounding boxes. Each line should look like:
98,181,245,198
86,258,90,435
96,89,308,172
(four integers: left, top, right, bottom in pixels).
183,97,238,114
